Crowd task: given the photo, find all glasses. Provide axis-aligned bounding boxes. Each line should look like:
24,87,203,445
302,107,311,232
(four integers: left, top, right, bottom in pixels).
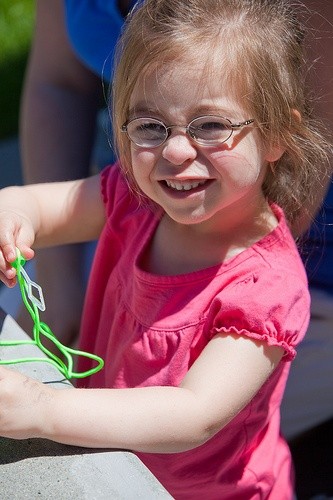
120,114,255,147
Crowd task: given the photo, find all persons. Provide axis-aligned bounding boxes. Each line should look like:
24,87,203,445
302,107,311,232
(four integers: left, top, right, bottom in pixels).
1,1,332,499
0,0,333,443
